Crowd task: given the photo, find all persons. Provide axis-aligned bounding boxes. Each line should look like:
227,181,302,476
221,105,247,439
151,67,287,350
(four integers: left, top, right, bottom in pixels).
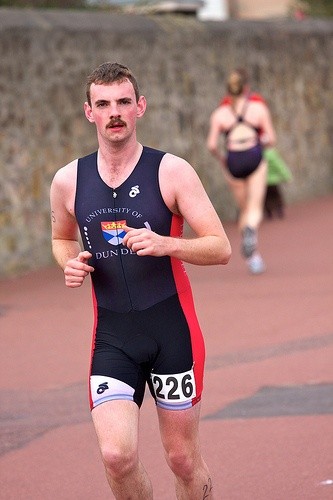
205,68,277,276
49,60,234,500
261,142,293,219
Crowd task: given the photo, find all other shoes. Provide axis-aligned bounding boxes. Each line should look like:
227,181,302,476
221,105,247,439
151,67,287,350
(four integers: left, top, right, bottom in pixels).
237,225,266,275
